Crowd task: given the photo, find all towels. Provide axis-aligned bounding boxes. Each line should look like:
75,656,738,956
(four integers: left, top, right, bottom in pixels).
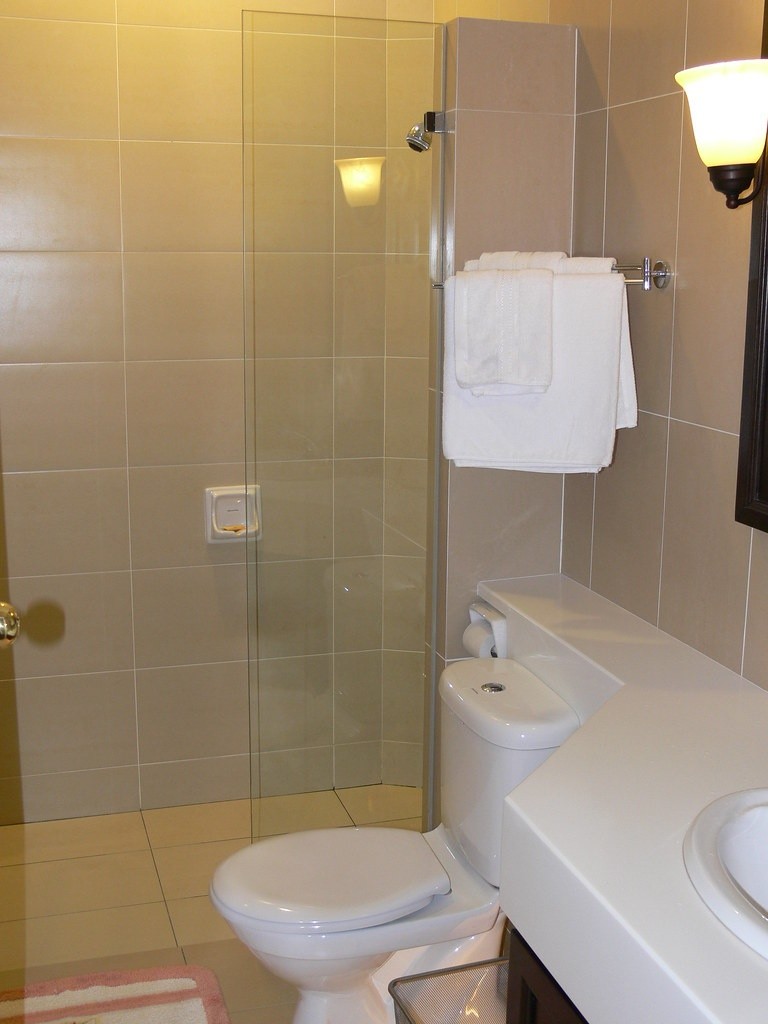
445,250,638,474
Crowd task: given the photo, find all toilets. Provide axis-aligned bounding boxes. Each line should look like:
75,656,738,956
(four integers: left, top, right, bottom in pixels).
211,656,578,1024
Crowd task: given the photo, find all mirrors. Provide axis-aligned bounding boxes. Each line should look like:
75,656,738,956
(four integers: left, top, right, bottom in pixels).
736,0,768,532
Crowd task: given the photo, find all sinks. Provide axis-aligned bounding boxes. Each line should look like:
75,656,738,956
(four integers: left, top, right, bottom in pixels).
681,787,767,962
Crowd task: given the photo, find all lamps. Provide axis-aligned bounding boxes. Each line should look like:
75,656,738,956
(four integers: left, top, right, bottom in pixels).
674,59,768,210
334,157,386,215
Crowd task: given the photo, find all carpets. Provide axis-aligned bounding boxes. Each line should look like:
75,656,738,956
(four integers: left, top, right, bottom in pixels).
0,965,232,1024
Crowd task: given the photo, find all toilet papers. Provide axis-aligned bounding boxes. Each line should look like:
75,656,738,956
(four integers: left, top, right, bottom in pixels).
460,620,496,659
